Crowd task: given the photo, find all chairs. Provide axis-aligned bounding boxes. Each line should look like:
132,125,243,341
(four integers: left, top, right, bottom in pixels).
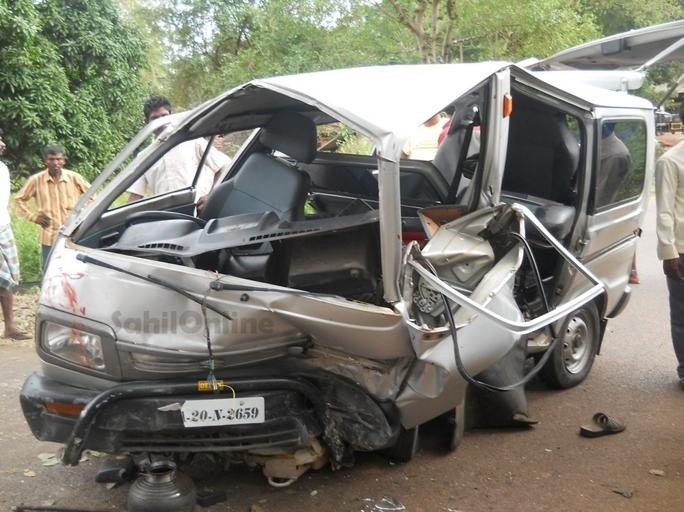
198,113,317,251
502,106,576,240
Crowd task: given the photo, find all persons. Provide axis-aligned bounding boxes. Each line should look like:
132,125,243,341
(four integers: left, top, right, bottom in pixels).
13,144,96,271
595,122,633,209
125,94,233,217
655,104,684,386
401,114,449,162
0,129,34,340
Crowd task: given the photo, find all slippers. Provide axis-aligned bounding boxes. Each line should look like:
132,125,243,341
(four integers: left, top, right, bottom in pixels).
580,408,625,438
4,326,31,339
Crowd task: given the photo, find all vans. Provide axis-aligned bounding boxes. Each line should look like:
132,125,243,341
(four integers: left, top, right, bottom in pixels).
18,60,658,473
